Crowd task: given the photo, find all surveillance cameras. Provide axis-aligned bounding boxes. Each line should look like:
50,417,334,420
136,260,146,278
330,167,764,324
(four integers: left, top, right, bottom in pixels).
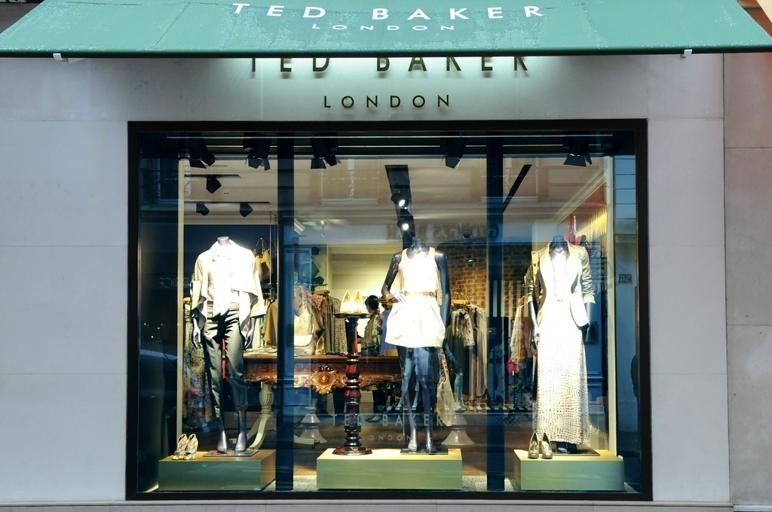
293,218,305,236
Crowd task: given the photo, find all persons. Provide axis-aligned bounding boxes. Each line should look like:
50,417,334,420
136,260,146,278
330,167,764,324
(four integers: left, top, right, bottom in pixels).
381,238,452,453
190,236,269,454
524,234,597,454
356,295,386,423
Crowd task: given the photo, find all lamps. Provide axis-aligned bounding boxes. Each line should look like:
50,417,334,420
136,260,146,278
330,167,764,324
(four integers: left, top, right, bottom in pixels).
184,141,342,218
390,189,410,231
445,156,461,169
564,153,592,167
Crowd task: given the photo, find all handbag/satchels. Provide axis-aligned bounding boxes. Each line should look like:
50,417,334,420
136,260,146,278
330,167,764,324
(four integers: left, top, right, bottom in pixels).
436,265,443,307
570,263,589,328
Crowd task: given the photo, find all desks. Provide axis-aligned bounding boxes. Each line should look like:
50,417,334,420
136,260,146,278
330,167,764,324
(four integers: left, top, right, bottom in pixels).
243,352,404,453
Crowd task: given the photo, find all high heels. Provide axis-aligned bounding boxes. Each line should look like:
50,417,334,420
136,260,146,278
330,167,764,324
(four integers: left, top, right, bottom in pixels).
541,433,553,459
527,433,540,460
365,402,535,423
183,433,198,460
172,434,187,460
